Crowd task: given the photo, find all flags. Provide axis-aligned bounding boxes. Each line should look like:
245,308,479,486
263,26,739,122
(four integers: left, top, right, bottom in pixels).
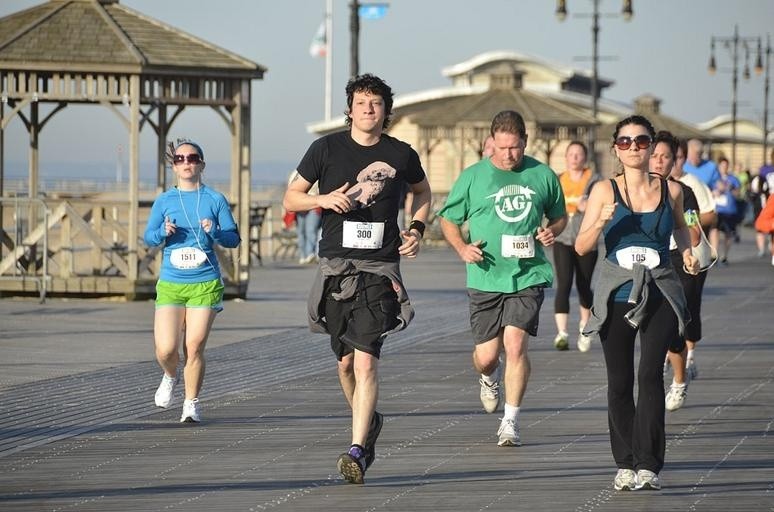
309,20,327,58
359,5,386,20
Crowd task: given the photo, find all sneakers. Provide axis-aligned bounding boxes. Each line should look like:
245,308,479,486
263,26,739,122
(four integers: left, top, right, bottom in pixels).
366,412,383,468
663,352,696,411
154,368,180,408
180,398,200,422
497,418,521,447
577,327,590,352
337,446,366,484
479,378,500,413
613,469,661,491
554,331,568,350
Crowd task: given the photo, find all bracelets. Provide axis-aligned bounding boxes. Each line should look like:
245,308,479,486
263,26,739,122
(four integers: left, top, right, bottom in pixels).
408,221,425,237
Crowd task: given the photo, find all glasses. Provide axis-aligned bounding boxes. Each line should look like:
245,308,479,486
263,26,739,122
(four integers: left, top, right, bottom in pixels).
616,135,653,150
174,154,201,164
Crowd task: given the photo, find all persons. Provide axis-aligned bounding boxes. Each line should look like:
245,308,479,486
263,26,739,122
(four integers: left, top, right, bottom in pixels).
576,116,703,491
437,109,569,446
649,131,774,279
554,140,601,352
143,142,242,424
282,73,432,484
480,133,496,160
284,167,319,267
643,128,712,412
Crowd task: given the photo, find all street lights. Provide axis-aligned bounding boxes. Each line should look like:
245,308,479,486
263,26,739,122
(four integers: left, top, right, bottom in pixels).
551,1,635,118
704,22,765,165
